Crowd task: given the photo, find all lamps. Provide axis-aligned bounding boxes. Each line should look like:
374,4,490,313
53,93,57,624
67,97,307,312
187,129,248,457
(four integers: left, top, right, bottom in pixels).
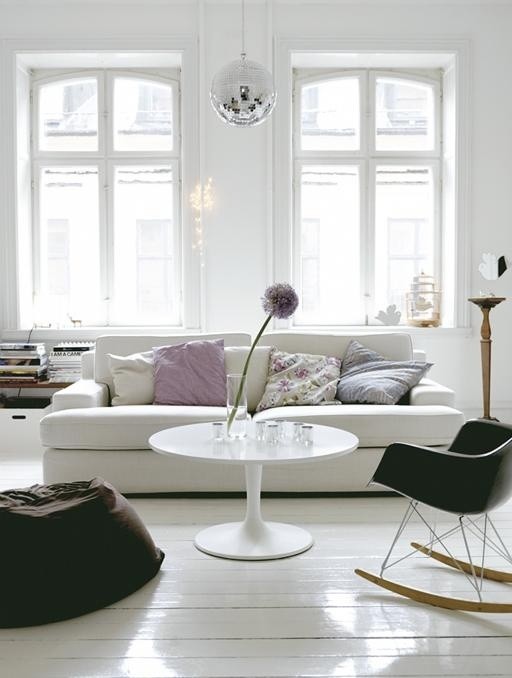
208,0,279,133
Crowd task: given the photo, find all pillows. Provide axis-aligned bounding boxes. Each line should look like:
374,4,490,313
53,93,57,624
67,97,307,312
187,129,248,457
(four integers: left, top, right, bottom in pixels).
152,337,228,408
336,339,434,406
224,345,273,410
255,346,342,413
105,351,157,407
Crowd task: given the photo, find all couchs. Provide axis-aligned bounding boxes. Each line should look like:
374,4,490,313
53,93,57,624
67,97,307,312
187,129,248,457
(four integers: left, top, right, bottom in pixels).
39,331,470,494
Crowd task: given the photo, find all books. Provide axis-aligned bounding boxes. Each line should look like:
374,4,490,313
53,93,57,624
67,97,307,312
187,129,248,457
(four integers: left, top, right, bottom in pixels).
1,341,47,382
48,341,94,381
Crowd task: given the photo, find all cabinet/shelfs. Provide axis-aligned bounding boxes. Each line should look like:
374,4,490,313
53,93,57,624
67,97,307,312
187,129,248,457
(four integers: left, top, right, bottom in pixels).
1,381,75,410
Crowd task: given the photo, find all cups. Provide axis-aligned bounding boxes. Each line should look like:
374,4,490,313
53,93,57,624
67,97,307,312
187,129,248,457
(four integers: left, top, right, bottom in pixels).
226,373,248,440
301,425,313,448
266,425,279,447
253,421,267,443
293,423,304,444
275,419,288,445
211,422,223,442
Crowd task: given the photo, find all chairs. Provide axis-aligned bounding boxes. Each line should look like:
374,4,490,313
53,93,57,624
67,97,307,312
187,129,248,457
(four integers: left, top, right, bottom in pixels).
353,419,512,616
